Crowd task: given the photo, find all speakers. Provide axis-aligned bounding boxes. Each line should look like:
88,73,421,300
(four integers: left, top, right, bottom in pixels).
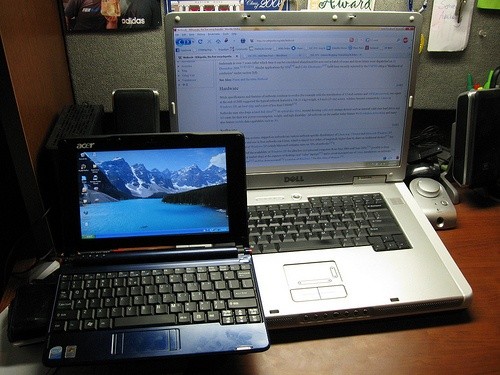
109,88,162,150
439,85,500,202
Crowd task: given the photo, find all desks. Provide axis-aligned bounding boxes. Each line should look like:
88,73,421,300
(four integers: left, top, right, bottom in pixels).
0,181,500,375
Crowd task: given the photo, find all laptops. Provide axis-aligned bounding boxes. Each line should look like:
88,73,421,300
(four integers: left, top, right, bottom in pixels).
41,130,270,366
164,11,475,330
